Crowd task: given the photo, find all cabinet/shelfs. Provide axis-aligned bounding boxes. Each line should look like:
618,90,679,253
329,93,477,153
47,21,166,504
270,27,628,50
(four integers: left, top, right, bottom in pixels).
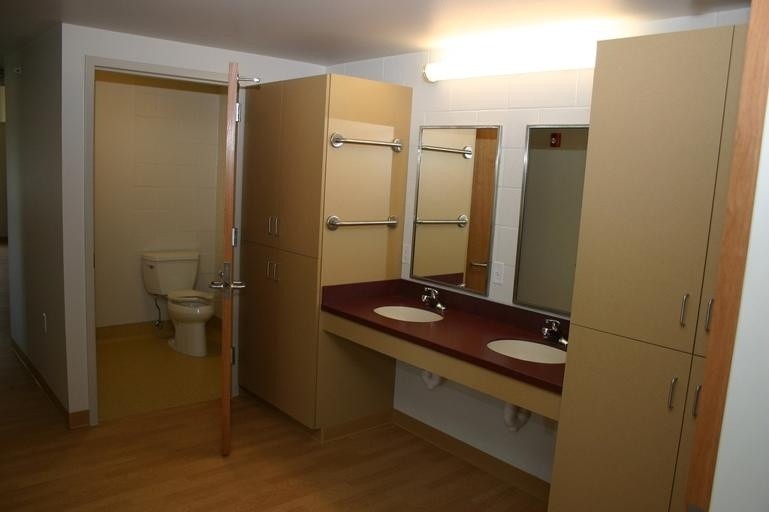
548,323,712,511
568,25,750,356
243,75,327,259
240,238,318,432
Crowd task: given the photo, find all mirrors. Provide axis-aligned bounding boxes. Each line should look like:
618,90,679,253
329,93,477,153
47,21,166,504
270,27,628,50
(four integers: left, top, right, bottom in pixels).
405,126,504,298
513,122,589,316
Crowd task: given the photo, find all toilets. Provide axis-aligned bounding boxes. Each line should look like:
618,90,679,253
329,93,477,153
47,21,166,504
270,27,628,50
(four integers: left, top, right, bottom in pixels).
142,251,214,357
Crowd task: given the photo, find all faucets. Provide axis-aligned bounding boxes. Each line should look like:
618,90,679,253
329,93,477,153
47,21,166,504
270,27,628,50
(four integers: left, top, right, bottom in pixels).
422,288,445,313
456,283,466,288
542,319,560,339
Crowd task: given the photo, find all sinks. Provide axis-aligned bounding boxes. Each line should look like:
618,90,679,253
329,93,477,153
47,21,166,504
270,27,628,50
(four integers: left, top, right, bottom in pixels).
487,338,567,365
374,304,443,323
